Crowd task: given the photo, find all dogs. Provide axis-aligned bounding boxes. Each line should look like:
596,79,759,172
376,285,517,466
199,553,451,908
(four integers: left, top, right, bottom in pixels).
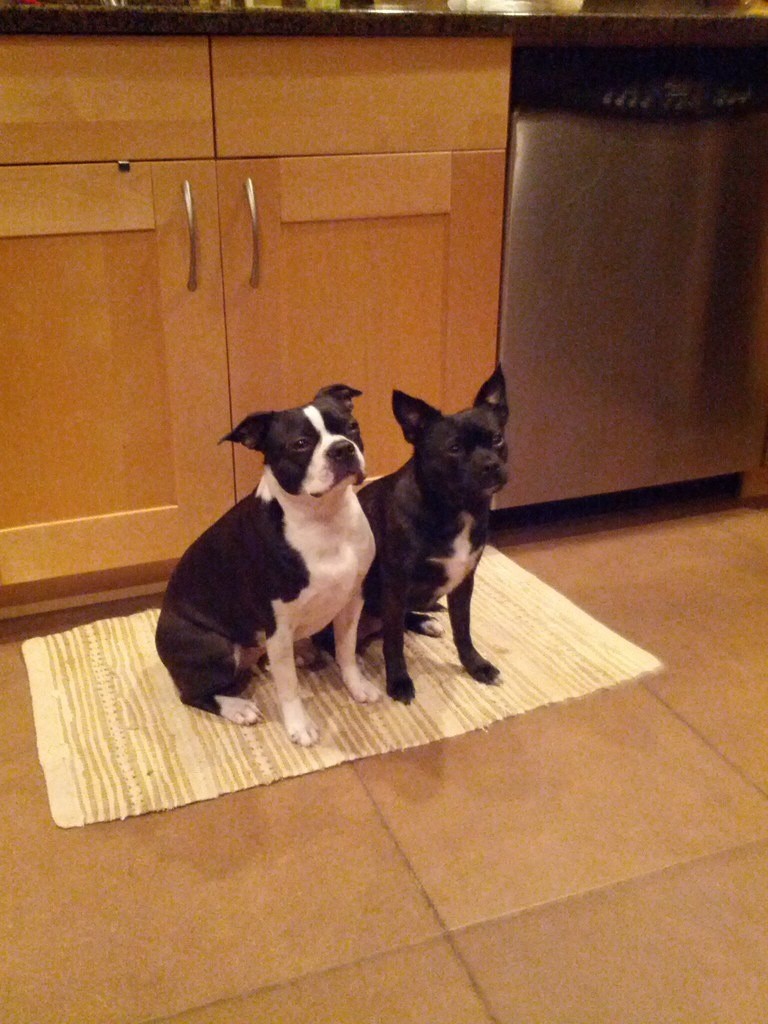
154,381,384,748
309,362,512,706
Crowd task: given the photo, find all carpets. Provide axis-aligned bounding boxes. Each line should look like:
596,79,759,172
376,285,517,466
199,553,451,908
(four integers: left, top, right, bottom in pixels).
22,538,667,833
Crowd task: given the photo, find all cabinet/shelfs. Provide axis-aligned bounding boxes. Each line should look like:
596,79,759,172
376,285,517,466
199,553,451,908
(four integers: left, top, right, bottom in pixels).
0,30,520,620
